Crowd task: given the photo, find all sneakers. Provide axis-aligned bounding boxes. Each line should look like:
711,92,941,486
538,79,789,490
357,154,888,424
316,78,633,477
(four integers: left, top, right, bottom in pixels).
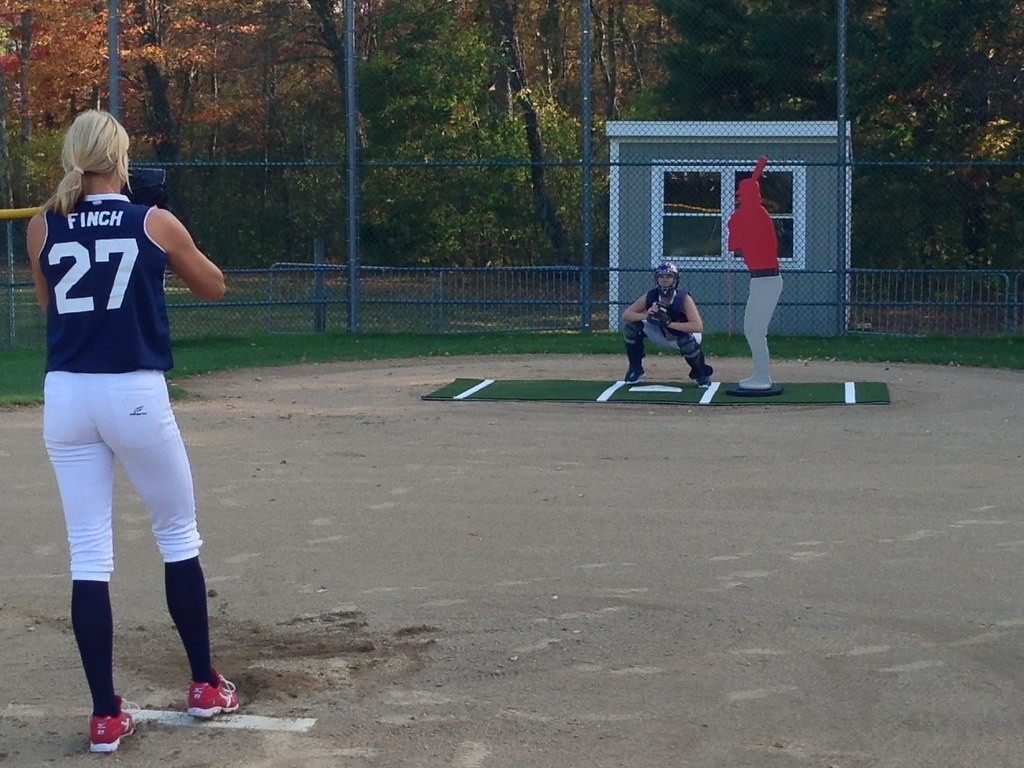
188,667,240,718
624,366,646,385
697,375,711,388
89,696,135,752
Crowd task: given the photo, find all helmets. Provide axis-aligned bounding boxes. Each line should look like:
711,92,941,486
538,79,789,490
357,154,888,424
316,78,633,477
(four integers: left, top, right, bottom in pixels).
655,262,680,296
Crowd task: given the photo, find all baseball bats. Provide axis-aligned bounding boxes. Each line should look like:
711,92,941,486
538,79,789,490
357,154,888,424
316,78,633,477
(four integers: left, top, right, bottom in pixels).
752,156,767,181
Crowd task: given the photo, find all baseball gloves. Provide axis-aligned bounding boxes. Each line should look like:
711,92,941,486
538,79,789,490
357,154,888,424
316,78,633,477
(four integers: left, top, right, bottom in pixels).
646,301,673,329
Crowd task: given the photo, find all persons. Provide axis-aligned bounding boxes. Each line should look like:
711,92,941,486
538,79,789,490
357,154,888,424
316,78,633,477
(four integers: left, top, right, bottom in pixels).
25,108,239,752
622,262,714,388
